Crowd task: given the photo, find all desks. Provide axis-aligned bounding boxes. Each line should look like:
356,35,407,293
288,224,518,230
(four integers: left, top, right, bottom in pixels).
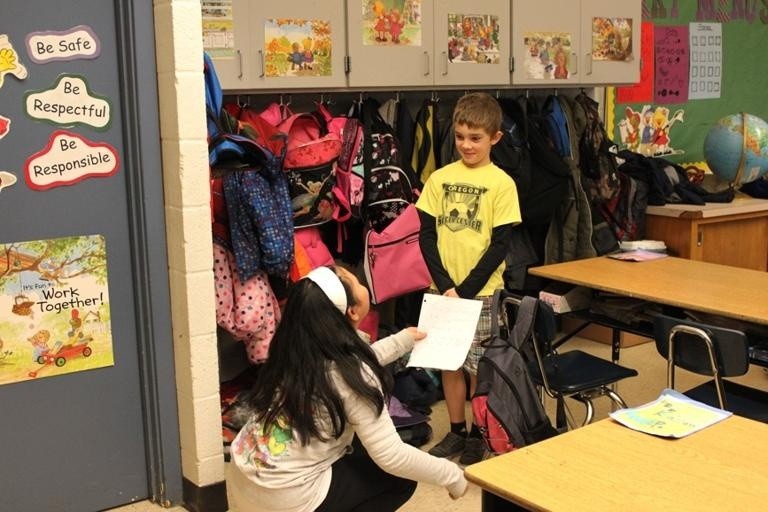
528,251,768,412
461,415,768,512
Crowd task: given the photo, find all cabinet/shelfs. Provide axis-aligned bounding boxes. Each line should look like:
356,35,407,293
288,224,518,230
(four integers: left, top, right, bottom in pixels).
512,2,642,92
645,191,768,273
347,2,512,93
200,2,347,93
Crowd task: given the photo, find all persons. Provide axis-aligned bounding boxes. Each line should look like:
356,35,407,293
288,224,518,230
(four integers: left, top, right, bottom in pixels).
412,92,525,467
223,265,469,509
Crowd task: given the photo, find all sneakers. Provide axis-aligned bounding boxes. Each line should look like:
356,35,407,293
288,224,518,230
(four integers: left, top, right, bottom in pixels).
427,432,466,457
459,432,487,465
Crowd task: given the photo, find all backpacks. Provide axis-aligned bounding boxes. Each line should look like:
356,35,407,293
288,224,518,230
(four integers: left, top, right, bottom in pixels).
617,146,735,207
470,289,560,456
208,92,623,306
383,350,445,411
378,387,432,446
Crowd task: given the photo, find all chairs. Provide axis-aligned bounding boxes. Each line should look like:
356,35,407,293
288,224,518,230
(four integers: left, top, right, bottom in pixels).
491,291,768,429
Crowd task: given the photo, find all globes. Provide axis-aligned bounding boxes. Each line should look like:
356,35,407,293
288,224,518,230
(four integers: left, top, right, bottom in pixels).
703,112,768,201
